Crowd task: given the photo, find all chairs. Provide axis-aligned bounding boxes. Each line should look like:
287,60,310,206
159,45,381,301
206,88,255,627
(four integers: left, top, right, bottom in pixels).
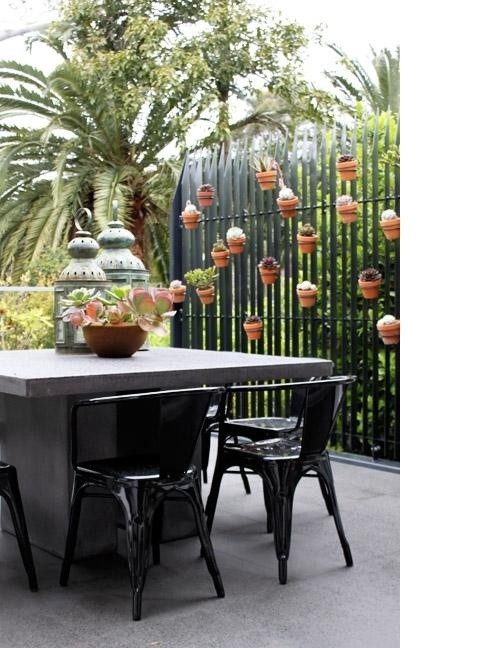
208,376,323,534
57,383,227,620
1,462,39,592
200,375,358,584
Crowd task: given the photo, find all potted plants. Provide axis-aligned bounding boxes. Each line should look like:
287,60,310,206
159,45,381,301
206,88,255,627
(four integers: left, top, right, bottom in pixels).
58,276,178,359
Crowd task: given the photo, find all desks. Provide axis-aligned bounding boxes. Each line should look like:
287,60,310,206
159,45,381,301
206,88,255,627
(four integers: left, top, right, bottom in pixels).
0,346,334,559
